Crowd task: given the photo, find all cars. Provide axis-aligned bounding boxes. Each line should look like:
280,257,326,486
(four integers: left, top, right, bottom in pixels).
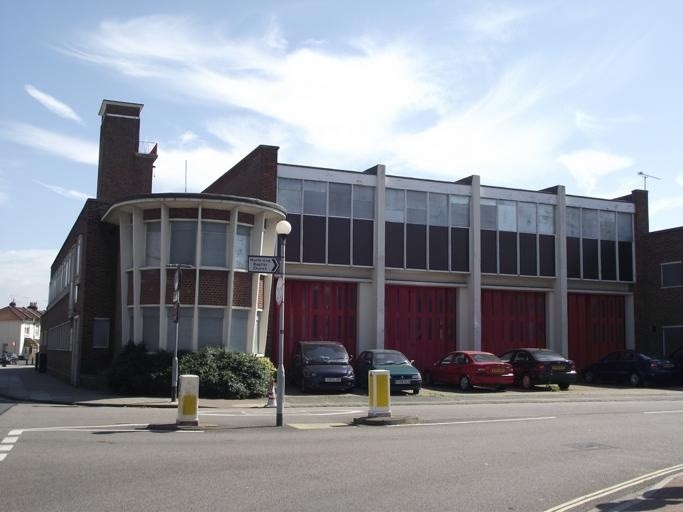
579,349,681,386
494,348,577,390
351,348,422,394
0,351,19,365
418,350,514,392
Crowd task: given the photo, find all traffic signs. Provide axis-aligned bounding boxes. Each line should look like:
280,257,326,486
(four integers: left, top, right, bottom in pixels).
248,254,280,274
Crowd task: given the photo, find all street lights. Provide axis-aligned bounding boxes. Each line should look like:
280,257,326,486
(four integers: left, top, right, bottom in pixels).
275,221,291,426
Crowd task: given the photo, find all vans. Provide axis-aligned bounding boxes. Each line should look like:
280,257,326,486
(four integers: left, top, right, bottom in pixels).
291,340,355,393
